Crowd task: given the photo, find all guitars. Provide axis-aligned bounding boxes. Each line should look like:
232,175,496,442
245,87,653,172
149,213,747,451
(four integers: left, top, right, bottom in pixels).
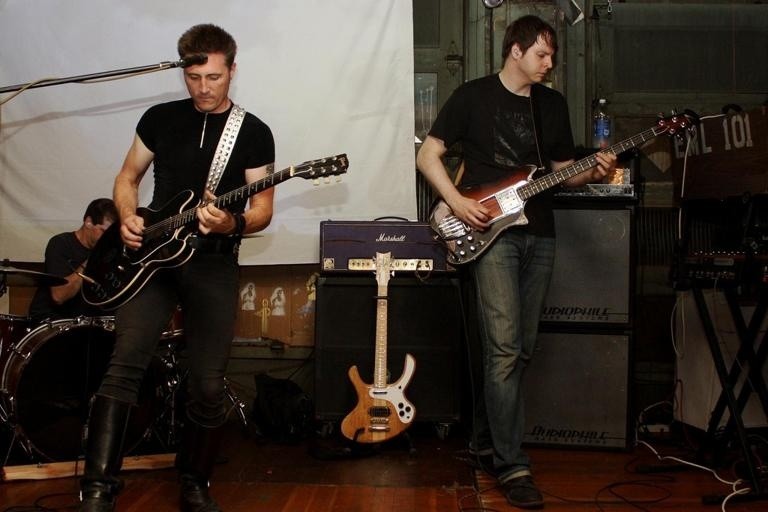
342,253,416,443
80,153,348,307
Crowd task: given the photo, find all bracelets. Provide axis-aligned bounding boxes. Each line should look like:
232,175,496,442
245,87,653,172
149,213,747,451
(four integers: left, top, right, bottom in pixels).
79,264,86,271
232,213,245,238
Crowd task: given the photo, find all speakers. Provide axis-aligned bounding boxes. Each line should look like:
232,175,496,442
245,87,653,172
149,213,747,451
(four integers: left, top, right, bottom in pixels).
520,330,634,454
532,204,635,330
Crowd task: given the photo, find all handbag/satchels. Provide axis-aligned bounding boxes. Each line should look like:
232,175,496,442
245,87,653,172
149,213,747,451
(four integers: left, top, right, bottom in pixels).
250,371,314,446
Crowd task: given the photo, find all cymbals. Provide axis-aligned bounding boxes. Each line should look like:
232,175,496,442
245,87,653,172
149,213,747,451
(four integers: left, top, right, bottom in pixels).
1,266,68,288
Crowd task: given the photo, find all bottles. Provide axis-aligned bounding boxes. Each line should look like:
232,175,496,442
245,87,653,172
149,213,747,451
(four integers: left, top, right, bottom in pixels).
592,98,613,147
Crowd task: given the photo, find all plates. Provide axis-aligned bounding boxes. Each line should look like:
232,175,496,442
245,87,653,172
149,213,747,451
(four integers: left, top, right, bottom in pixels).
586,183,635,195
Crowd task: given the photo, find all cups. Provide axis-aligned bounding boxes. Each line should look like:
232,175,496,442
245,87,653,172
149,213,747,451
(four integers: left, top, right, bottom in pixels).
603,168,630,185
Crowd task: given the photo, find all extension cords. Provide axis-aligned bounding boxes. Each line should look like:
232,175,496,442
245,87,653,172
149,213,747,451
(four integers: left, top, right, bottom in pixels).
639,424,670,433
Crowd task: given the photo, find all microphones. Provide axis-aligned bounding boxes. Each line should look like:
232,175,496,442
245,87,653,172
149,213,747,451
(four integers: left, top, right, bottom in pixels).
178,52,208,68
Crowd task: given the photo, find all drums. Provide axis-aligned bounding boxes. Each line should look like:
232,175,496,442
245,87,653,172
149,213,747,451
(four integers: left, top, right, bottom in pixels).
3,316,177,461
429,107,695,267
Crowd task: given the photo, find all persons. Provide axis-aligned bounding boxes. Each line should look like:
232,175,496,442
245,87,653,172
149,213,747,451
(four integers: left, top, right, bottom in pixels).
28,199,117,314
416,15,617,509
79,23,276,512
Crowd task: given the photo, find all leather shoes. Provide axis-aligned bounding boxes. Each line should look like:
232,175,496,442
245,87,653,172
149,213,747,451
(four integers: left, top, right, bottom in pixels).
468,451,533,471
500,476,543,508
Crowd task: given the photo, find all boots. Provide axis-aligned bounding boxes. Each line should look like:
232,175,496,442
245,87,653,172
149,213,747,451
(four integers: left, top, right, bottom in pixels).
77,391,139,512
185,405,230,511
